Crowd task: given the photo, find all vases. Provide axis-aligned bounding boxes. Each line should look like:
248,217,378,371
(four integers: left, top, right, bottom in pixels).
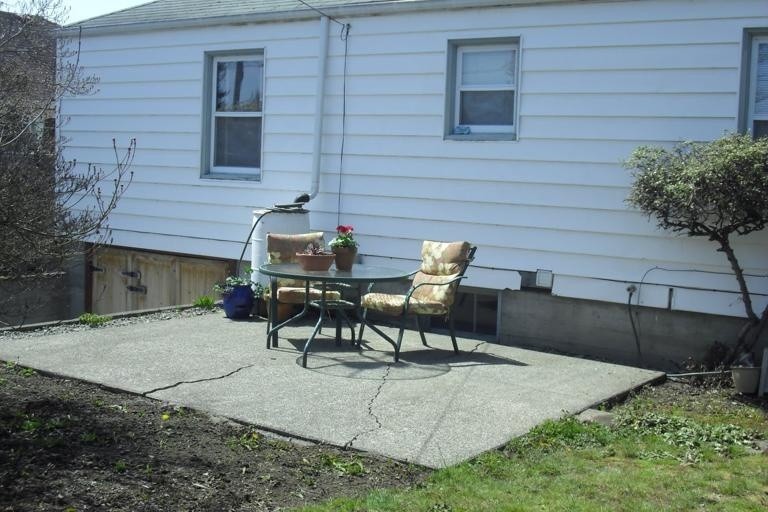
729,366,762,393
331,247,358,271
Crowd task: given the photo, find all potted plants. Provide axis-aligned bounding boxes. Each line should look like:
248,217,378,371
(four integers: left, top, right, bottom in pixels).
295,240,336,272
211,265,265,321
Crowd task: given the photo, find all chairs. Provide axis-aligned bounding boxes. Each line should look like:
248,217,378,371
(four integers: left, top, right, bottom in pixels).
356,238,477,363
265,231,342,346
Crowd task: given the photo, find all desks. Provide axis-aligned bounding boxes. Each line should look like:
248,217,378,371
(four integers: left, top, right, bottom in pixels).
256,262,410,369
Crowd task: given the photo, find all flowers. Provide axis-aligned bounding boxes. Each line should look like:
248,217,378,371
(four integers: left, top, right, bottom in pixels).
327,223,360,249
